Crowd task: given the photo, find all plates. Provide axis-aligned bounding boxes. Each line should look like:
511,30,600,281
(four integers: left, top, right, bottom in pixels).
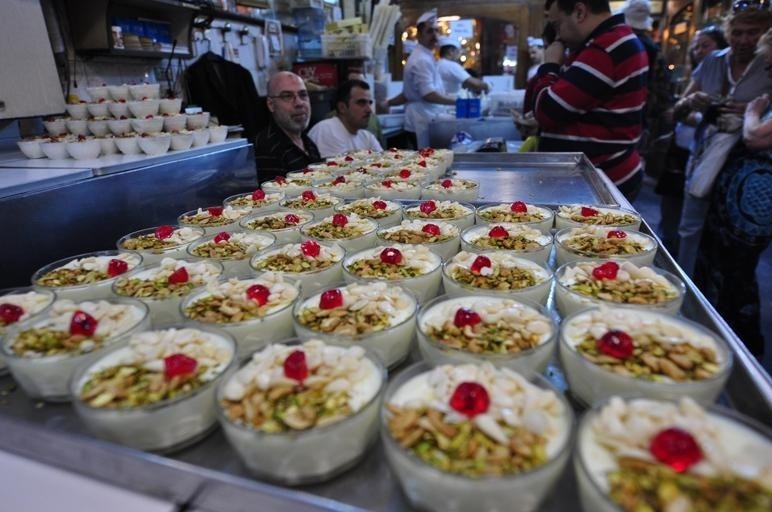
319,33,373,59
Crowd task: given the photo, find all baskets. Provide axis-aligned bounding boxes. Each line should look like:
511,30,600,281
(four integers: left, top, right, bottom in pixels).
321,33,372,61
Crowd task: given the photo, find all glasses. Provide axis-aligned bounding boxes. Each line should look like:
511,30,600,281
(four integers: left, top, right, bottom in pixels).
270,93,308,103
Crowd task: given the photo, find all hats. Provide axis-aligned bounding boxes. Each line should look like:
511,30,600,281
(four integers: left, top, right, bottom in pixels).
611,0,653,29
438,36,462,50
526,36,544,47
417,9,438,29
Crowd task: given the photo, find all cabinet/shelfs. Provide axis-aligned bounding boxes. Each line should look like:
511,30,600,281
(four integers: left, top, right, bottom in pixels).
72,0,200,58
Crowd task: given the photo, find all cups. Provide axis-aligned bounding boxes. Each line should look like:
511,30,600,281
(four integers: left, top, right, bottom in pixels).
455,97,481,119
112,25,125,50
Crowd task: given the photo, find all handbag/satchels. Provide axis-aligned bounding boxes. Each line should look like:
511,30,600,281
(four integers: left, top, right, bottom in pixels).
689,132,740,198
644,132,677,181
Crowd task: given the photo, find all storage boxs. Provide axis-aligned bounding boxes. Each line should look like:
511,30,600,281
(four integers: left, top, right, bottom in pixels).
320,15,373,59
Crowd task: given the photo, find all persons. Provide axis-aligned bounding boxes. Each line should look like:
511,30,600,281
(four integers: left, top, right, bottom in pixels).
248,69,320,186
402,10,458,149
614,0,663,120
519,23,568,142
524,35,546,83
652,22,730,274
377,89,410,115
434,35,489,95
672,7,772,357
742,25,772,378
509,0,651,205
307,78,385,162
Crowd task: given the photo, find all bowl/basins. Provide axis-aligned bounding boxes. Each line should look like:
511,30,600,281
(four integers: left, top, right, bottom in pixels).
1,144,770,509
15,85,229,160
